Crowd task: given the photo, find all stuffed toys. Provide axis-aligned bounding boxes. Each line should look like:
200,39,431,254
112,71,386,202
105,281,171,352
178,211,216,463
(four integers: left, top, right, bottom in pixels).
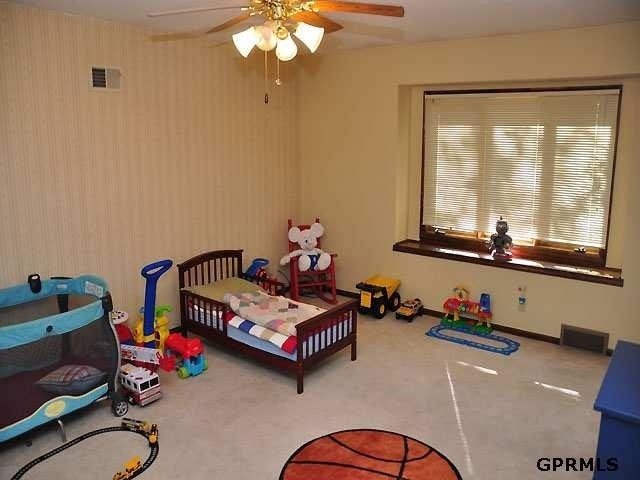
489,220,512,261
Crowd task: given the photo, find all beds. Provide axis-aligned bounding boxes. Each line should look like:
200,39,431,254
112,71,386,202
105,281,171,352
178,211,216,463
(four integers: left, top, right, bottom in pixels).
0,273,129,444
175,250,358,394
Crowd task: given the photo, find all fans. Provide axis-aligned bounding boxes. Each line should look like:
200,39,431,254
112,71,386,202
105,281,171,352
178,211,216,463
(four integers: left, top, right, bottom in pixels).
141,0,404,103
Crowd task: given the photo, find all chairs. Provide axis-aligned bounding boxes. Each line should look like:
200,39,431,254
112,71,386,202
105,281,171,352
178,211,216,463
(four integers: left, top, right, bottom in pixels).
286,219,339,307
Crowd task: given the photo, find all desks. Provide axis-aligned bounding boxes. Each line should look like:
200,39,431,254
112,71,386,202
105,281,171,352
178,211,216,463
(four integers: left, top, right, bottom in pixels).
594,339,638,478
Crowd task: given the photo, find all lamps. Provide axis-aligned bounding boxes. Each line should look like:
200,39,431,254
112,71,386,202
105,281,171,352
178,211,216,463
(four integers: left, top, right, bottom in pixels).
232,16,325,62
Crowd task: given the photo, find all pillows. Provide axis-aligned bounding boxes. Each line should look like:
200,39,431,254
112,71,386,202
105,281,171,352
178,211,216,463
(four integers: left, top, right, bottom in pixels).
35,362,108,397
